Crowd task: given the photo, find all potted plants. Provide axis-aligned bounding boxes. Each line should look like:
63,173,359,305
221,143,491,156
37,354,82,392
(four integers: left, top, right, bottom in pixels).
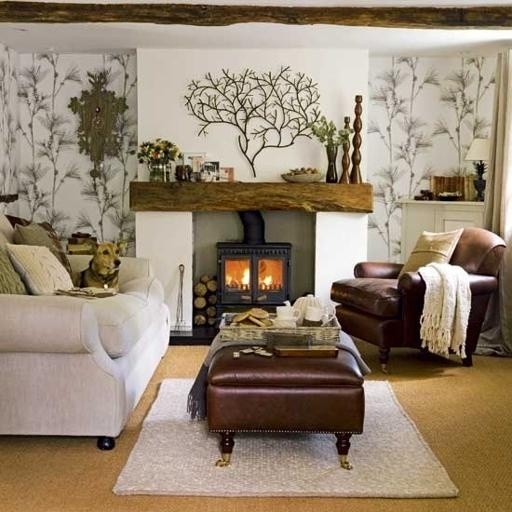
313,117,351,182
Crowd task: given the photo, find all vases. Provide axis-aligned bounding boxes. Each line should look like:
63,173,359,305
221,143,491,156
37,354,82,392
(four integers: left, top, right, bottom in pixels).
148,164,164,184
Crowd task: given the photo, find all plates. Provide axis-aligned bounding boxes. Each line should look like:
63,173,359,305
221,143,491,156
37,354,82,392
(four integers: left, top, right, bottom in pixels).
436,194,462,201
282,173,324,184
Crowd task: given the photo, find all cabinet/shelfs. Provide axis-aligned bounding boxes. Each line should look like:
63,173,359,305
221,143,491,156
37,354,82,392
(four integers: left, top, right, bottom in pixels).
394,197,485,265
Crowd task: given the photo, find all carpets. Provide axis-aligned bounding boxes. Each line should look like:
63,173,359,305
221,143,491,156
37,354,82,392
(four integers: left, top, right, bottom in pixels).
111,377,461,502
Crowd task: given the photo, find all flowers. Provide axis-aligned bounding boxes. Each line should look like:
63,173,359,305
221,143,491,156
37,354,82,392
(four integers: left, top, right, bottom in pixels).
138,137,183,168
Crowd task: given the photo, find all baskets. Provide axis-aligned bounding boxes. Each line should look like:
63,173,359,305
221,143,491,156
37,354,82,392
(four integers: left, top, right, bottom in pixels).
219,312,340,344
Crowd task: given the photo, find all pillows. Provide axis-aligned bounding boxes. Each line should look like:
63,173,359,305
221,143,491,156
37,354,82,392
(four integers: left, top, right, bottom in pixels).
1,234,30,292
6,243,74,295
399,228,463,278
9,224,65,265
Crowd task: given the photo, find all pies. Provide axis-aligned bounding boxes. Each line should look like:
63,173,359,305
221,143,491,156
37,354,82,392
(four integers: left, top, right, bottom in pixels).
229,308,272,328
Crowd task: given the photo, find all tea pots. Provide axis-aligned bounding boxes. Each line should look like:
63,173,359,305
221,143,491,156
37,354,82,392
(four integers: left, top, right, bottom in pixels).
284,294,336,325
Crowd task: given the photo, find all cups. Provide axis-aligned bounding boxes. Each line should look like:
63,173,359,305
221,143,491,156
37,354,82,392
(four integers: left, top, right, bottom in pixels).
304,307,328,326
277,306,301,322
175,163,217,183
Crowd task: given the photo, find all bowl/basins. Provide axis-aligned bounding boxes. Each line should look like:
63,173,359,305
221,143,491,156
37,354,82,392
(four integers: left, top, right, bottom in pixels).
67,238,86,244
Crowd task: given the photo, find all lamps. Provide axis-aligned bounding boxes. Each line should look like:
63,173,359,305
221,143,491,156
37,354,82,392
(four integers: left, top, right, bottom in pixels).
464,138,491,201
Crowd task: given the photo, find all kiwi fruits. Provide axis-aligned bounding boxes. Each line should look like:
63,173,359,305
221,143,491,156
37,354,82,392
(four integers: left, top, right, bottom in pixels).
287,167,318,176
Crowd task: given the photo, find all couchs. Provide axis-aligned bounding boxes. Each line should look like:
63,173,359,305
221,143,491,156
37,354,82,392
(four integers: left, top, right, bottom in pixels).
1,210,172,451
329,222,507,372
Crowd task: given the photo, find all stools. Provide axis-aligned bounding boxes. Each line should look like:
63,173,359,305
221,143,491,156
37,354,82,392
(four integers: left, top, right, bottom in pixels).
203,306,365,469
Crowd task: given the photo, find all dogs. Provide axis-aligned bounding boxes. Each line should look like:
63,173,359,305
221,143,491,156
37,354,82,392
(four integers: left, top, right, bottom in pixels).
73,239,125,290
